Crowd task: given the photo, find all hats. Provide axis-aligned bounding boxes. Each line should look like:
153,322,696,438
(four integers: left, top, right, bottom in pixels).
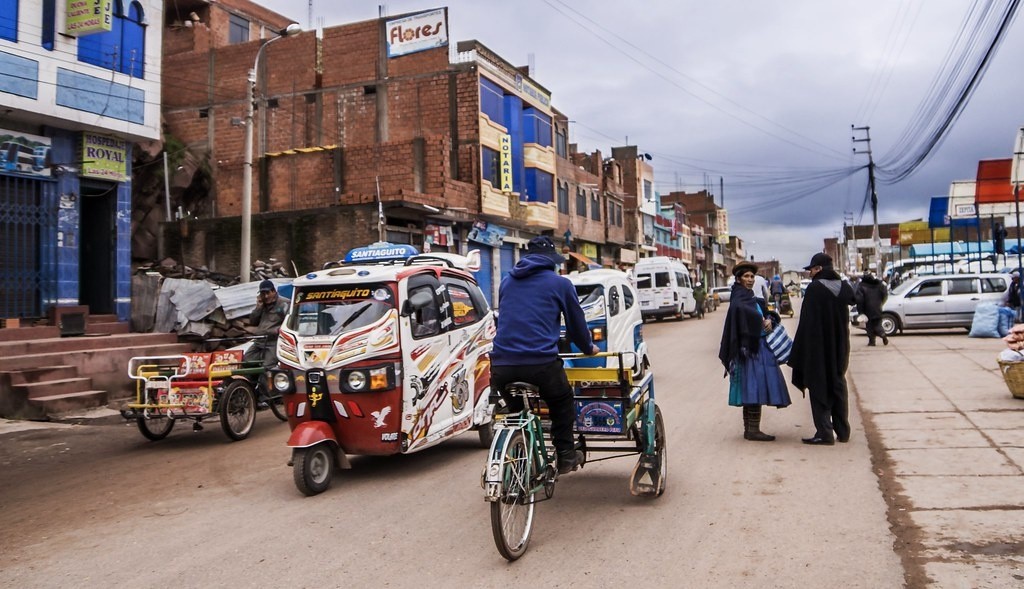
256,280,274,293
803,253,833,269
696,281,702,287
863,268,872,276
773,274,780,280
732,261,758,277
1011,271,1020,278
526,236,565,263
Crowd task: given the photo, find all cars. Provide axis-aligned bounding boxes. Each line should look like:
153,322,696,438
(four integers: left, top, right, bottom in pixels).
851,274,1013,338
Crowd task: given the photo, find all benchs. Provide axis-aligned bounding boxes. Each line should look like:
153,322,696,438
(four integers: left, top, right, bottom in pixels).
563,352,642,387
155,350,244,415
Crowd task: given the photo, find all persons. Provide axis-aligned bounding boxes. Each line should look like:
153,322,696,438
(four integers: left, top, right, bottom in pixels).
241,280,291,410
787,252,851,446
890,271,914,290
488,237,600,475
857,267,889,346
718,261,793,441
1003,270,1024,324
993,222,1008,254
752,274,786,312
693,282,707,320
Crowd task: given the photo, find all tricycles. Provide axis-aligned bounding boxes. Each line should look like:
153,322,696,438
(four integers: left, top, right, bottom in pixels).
768,293,794,316
479,350,668,560
122,333,288,442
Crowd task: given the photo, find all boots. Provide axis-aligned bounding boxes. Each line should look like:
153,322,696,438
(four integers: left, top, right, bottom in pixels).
743,407,750,438
747,411,776,440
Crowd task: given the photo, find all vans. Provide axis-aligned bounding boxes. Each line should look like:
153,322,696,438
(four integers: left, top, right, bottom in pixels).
635,257,699,319
709,286,733,303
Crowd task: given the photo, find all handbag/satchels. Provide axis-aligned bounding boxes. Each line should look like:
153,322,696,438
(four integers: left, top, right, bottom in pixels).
764,319,793,365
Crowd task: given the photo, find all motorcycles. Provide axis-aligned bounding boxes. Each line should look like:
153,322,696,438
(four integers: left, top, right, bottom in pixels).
564,269,650,375
272,241,497,497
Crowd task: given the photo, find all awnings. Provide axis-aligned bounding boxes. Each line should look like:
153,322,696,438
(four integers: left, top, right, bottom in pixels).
569,251,604,270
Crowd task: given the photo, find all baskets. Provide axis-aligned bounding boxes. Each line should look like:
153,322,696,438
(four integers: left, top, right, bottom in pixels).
998,358,1024,398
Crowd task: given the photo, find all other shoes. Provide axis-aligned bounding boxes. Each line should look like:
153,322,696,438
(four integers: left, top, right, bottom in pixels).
802,437,835,445
836,437,847,443
882,336,888,345
868,343,876,346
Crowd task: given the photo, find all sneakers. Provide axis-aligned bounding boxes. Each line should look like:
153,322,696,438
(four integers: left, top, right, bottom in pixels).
557,450,584,473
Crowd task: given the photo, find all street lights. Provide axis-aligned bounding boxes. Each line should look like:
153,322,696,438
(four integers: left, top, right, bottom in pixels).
241,22,304,281
